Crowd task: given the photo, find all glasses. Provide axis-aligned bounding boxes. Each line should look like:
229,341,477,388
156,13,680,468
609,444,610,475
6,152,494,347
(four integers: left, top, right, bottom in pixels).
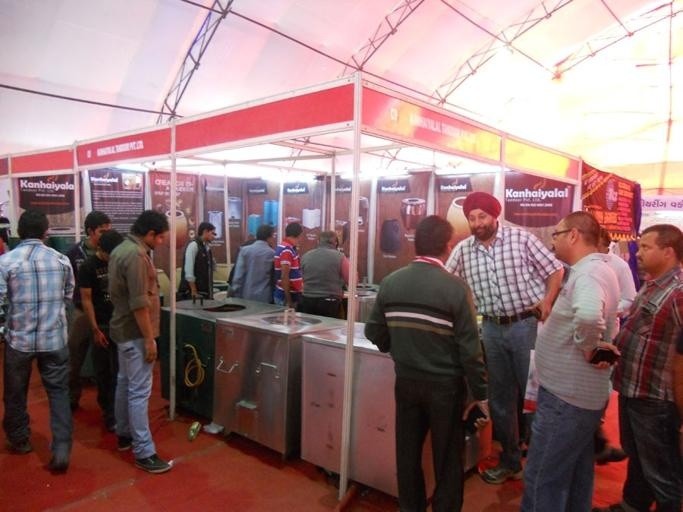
549,229,577,237
209,231,217,237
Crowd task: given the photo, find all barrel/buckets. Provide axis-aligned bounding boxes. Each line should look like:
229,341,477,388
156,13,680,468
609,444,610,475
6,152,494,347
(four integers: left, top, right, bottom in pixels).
249,214,261,236
401,198,426,241
302,208,321,239
208,211,225,246
348,196,368,232
165,209,188,248
379,217,403,259
262,201,278,226
445,197,471,234
228,196,242,228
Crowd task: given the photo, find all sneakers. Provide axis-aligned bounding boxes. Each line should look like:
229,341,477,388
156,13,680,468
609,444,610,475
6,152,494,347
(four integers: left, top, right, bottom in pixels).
118,436,135,451
47,445,69,474
484,458,524,483
7,438,33,454
104,421,120,431
135,453,172,473
592,446,625,464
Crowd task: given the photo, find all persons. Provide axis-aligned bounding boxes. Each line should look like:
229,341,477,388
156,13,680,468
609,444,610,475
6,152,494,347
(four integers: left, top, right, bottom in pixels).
107,210,171,475
176,222,217,302
519,211,620,512
364,216,489,512
595,227,637,464
228,224,276,302
445,192,565,485
69,229,124,434
299,231,350,317
606,224,683,512
273,222,303,309
0,207,75,474
64,211,113,411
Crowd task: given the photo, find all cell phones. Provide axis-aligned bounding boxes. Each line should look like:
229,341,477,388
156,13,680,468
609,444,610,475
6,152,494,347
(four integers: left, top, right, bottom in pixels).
530,309,540,320
465,405,486,433
589,348,621,365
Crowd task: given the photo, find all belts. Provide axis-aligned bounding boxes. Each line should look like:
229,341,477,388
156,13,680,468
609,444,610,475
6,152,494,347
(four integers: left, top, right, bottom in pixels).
481,312,537,324
